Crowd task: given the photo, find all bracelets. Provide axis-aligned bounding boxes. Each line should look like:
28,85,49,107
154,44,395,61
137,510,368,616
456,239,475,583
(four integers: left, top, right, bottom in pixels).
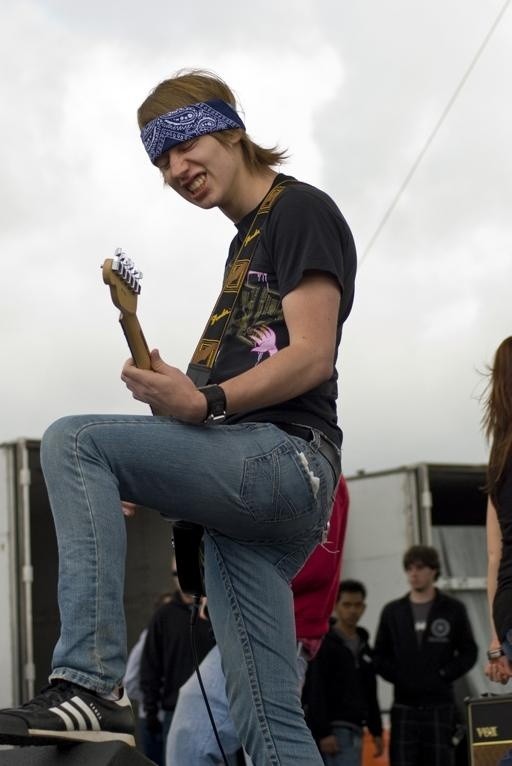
486,648,506,660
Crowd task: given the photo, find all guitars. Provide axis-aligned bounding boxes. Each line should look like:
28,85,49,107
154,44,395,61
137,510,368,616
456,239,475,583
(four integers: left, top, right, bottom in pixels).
101,247,207,597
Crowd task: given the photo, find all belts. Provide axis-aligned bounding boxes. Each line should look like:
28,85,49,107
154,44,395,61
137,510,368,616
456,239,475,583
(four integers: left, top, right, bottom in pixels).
274,423,342,485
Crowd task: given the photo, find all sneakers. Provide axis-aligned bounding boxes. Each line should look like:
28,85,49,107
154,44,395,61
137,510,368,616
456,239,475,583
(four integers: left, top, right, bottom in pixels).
0,675,137,749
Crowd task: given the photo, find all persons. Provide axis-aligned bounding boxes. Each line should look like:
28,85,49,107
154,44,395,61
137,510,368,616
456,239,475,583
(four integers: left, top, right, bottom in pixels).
166,472,349,766
0,74,357,766
373,544,479,766
482,336,512,766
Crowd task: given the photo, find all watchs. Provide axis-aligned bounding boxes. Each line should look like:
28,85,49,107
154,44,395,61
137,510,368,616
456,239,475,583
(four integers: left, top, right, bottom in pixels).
196,382,228,428
